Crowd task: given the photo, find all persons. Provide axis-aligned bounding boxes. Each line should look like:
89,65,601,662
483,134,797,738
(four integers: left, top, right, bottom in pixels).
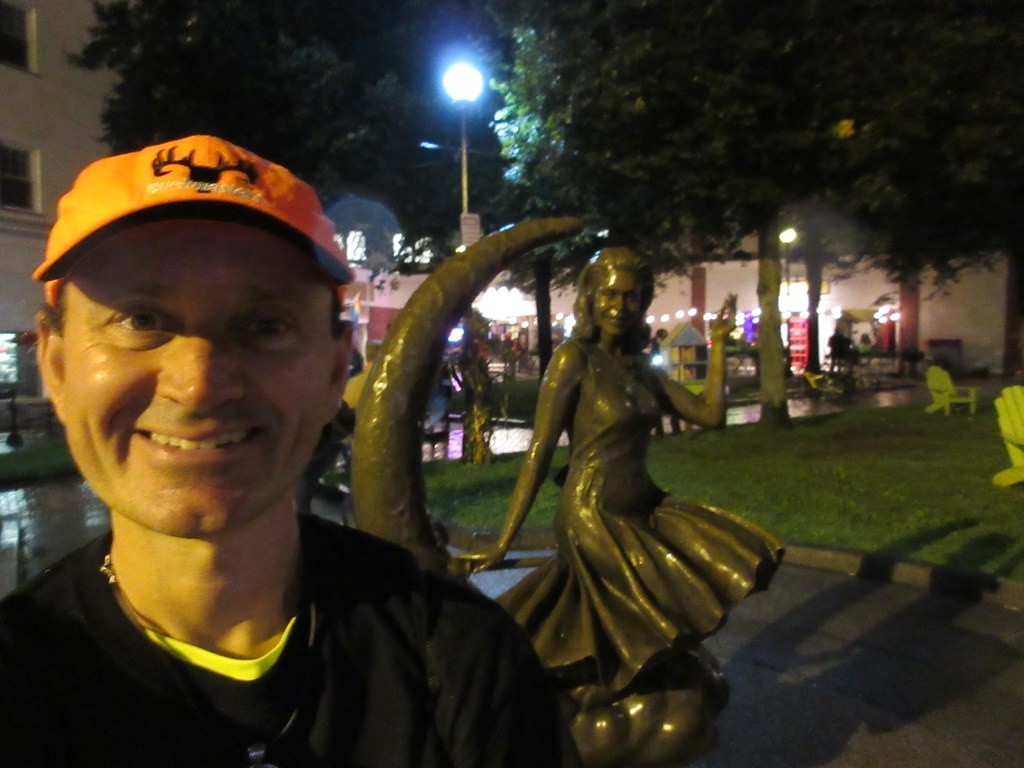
827,328,871,372
461,248,784,708
646,329,682,439
0,136,585,767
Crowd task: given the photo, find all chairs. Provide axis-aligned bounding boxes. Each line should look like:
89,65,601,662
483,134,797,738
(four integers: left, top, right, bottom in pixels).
990,386,1024,488
923,366,982,416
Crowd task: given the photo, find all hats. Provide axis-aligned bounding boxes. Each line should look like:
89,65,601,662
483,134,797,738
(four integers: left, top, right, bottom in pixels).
31,134,355,286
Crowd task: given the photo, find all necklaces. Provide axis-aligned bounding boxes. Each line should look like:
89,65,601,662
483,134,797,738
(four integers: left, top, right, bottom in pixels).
98,552,321,768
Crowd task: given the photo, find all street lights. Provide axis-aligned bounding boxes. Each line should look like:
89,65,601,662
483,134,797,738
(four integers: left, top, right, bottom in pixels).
421,140,553,378
779,227,798,379
443,63,485,254
